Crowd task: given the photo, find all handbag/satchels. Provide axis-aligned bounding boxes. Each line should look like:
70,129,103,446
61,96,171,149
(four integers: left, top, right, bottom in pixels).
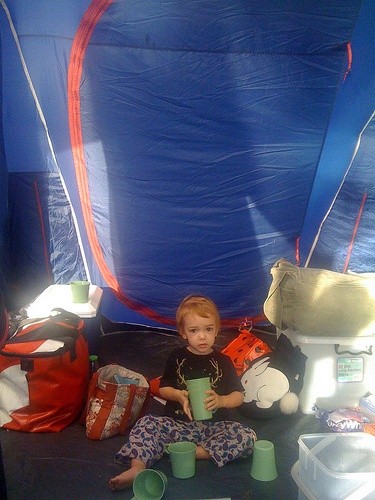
236,333,309,420
85,363,150,441
0,308,93,433
263,258,375,338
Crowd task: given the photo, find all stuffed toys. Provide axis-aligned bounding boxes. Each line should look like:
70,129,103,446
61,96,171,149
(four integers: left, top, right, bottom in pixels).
234,354,299,422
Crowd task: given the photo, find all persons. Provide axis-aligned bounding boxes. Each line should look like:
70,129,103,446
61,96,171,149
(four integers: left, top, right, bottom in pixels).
108,290,258,493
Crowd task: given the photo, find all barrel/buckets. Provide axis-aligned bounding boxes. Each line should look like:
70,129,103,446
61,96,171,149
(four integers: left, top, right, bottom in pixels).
291,432,375,500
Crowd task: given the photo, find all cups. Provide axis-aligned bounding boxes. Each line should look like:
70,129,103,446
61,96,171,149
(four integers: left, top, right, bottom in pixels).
186,376,212,420
70,281,90,303
250,439,278,481
163,441,196,479
129,469,168,500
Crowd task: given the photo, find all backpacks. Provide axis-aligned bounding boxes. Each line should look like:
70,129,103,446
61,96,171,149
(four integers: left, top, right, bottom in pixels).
221,329,273,377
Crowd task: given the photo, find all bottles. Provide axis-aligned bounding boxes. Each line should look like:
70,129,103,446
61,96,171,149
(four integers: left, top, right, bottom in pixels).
89,355,98,375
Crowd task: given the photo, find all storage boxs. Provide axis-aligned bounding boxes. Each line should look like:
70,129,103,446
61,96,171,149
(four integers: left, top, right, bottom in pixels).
273,325,375,415
